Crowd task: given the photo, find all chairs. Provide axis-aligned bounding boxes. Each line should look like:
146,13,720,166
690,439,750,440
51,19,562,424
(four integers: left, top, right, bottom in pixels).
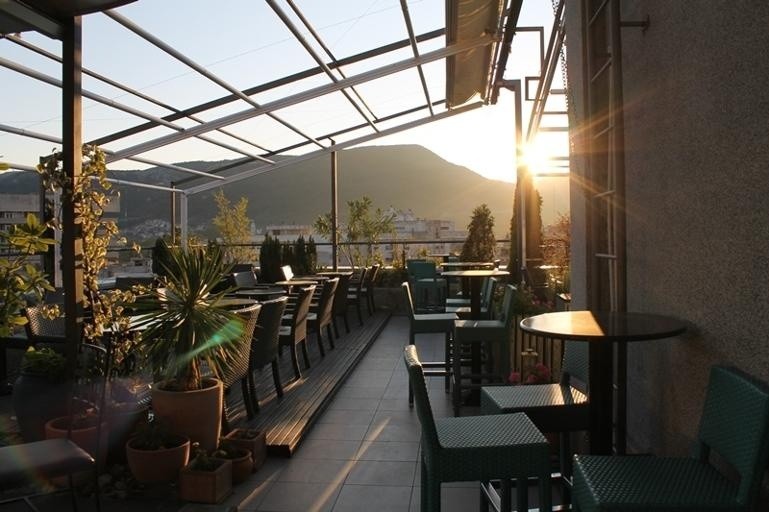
479,340,593,511
0,342,111,511
222,264,381,432
568,364,769,512
19,303,68,352
400,258,519,418
402,345,550,511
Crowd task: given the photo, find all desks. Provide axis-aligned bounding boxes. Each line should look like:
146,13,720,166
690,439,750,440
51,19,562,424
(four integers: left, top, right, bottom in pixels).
519,310,688,455
95,313,172,385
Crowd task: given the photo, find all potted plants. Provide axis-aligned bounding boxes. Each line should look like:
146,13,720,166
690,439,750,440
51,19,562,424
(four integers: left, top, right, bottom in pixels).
179,446,234,504
125,405,191,483
44,344,110,467
212,436,253,485
33,143,154,461
221,427,266,472
117,237,258,459
13,346,74,443
